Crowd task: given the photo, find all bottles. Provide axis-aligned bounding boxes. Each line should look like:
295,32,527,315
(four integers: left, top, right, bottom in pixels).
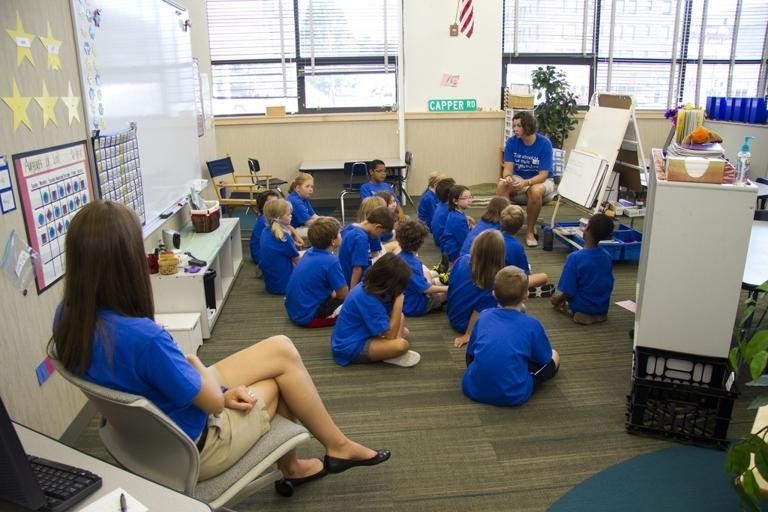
543,226,553,252
147,253,158,273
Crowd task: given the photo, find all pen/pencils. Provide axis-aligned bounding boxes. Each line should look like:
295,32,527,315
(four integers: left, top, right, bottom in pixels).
120,493,128,512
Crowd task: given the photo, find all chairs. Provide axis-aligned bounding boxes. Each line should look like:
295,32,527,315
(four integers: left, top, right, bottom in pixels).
245,158,288,215
46,354,309,512
203,153,272,240
337,161,375,227
509,191,558,242
755,178,768,210
384,152,418,214
754,210,768,221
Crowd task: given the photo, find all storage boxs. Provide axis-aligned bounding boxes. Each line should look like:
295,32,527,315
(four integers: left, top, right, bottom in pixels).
553,221,642,262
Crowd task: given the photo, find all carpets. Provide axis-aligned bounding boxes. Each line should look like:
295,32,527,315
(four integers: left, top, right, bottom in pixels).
219,207,336,239
545,439,768,512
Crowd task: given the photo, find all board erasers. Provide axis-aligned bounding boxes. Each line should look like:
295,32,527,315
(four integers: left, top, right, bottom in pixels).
160,209,173,219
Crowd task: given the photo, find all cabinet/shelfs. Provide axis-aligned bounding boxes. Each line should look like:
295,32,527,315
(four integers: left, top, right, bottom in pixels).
145,216,244,339
502,83,536,153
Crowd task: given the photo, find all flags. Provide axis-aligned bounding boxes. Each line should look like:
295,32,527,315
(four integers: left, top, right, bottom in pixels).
458,0,474,38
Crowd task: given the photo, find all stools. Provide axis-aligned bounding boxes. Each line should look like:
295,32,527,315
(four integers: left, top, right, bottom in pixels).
153,313,204,359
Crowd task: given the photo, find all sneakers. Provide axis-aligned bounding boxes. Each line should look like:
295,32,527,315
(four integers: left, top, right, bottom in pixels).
574,311,608,325
401,326,409,339
382,350,421,368
527,283,555,298
526,234,538,246
432,252,451,285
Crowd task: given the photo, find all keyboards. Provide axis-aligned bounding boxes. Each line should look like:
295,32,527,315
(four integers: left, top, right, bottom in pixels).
25,452,102,512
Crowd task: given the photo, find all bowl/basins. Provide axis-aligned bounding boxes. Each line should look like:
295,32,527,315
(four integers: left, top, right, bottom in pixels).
158,250,181,276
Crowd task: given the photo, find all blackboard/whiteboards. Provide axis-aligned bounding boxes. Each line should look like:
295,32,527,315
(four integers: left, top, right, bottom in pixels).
69,1,210,248
556,106,633,213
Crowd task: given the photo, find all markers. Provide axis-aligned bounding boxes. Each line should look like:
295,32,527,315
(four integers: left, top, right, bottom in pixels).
177,198,189,206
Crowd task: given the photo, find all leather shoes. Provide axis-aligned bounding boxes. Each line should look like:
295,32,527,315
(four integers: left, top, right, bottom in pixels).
324,449,391,473
284,455,329,486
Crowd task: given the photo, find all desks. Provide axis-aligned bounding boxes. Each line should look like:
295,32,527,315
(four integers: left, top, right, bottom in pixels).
632,148,759,385
499,146,506,179
752,180,768,195
737,219,768,347
299,160,407,207
10,421,214,512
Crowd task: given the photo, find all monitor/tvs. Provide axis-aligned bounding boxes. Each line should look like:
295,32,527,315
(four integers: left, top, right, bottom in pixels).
0,396,47,512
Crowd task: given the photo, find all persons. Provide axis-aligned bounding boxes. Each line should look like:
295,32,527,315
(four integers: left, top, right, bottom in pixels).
51,198,392,486
447,229,526,348
550,215,616,324
501,111,555,247
252,160,449,368
418,171,547,288
461,266,560,406
